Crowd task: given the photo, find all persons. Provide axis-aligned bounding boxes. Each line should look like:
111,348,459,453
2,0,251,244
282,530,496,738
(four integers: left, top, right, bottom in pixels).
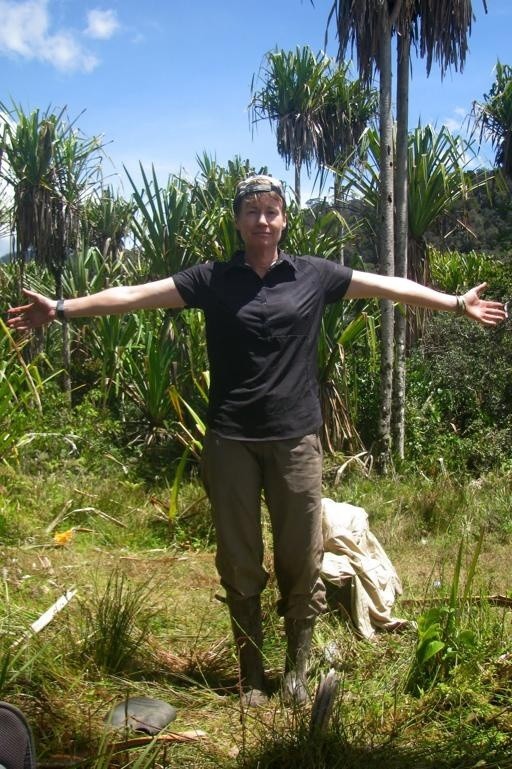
5,172,507,707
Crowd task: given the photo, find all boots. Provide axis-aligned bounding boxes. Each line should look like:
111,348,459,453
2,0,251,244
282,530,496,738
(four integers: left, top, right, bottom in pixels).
282,618,316,707
228,595,268,706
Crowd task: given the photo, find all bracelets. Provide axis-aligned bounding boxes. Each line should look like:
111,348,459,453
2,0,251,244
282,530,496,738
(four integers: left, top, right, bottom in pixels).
454,295,466,318
56,300,65,319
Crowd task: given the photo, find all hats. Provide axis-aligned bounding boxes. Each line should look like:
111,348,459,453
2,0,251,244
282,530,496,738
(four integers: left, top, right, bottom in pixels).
233,174,289,245
